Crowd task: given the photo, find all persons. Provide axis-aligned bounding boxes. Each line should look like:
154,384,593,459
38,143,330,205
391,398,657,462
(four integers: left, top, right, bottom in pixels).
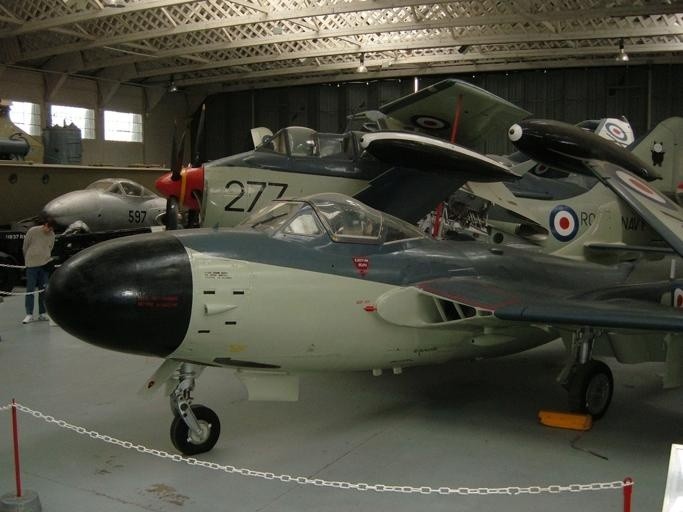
21,217,57,324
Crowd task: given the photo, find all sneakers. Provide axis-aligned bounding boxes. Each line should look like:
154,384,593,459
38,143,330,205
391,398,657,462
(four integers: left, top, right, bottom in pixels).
22,314,34,324
38,313,50,321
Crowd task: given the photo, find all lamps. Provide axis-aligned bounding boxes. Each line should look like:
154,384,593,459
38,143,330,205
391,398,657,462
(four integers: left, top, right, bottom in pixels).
354,53,368,74
165,74,177,95
615,39,629,63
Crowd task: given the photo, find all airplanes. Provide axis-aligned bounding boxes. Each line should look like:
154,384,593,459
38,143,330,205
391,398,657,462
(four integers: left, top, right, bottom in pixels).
0,74,682,460
